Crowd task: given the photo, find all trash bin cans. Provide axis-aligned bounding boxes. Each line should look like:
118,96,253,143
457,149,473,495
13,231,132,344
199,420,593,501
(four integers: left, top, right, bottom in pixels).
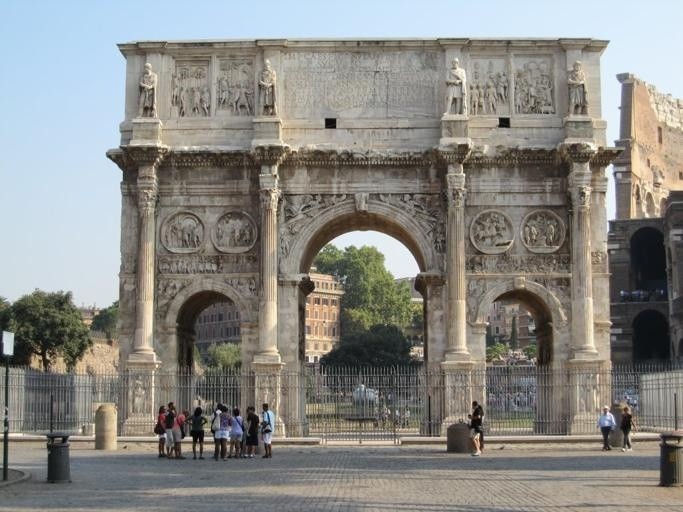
47,432,69,484
660,432,683,487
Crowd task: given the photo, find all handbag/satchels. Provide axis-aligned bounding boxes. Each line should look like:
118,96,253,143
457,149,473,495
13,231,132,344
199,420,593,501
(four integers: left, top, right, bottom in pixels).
212,416,220,430
262,423,272,433
180,425,185,438
154,422,165,434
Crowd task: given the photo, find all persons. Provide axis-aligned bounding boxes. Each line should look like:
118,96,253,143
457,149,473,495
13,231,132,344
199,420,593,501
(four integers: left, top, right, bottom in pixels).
157,401,275,460
470,70,554,115
442,57,467,116
524,221,554,247
488,388,536,412
138,63,158,119
566,60,584,115
619,407,638,451
172,65,255,118
476,405,484,449
596,405,617,451
256,59,278,117
373,405,411,428
619,287,666,304
468,402,483,456
165,222,200,248
217,215,251,249
